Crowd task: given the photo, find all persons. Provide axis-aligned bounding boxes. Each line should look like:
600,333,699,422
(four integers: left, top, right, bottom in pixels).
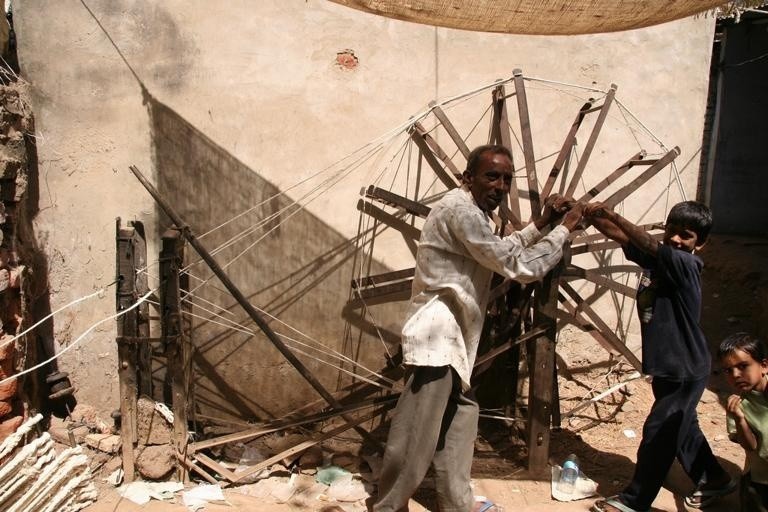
374,145,587,512
722,331,768,511
547,195,737,512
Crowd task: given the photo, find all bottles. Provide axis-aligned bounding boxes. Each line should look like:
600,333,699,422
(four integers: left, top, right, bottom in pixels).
556,453,580,495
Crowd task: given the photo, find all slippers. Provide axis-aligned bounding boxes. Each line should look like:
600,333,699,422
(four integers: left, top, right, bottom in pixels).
594,494,637,511
476,501,505,512
685,479,737,508
